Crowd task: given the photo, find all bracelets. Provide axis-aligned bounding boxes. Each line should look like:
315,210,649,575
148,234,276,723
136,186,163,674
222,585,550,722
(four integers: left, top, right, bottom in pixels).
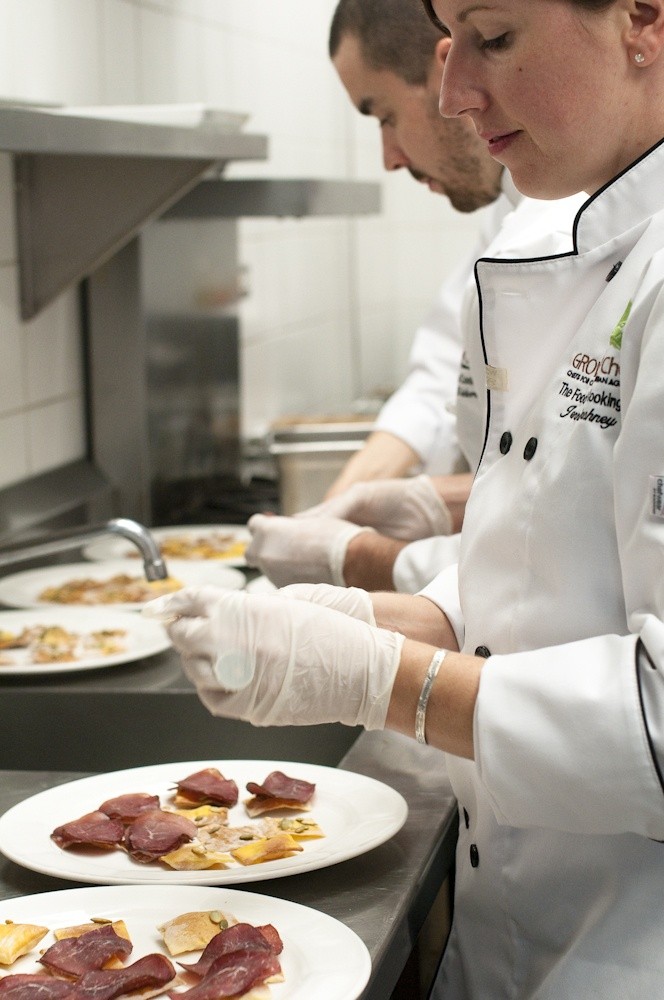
413,647,452,748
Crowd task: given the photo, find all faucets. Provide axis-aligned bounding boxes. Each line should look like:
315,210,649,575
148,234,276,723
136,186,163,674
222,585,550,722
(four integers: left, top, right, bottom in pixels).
2,517,170,585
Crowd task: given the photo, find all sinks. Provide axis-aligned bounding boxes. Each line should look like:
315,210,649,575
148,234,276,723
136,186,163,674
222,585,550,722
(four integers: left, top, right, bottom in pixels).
1,684,379,773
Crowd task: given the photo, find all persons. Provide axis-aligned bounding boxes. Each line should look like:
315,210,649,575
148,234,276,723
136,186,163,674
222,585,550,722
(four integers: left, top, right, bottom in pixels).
247,0,592,598
166,0,663,1000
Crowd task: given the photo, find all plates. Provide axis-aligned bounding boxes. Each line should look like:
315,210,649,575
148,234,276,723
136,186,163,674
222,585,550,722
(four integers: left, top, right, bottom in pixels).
0,601,173,677
85,519,260,572
0,559,247,609
1,884,372,998
1,757,410,883
